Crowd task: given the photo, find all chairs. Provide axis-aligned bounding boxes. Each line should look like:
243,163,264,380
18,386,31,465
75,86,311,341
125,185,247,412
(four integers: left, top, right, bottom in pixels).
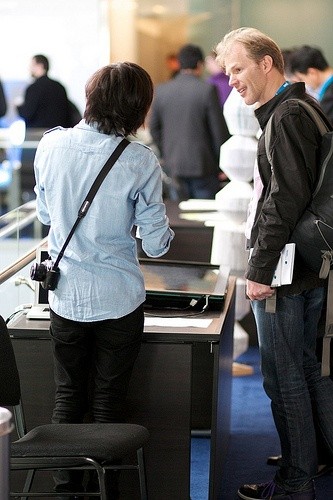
0,314,150,500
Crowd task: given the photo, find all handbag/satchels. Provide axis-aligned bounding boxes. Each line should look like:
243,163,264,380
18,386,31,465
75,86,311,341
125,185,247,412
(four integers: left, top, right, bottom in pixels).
264,101,333,279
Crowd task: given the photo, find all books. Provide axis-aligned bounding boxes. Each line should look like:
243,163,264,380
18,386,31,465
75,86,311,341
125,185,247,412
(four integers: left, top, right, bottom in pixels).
247,243,296,288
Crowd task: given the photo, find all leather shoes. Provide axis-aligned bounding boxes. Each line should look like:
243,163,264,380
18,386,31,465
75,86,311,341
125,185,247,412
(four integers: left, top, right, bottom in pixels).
268,455,283,465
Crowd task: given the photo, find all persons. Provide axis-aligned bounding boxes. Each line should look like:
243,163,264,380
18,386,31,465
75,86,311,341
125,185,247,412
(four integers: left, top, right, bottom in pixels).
13,53,68,130
214,27,333,499
34,61,175,423
148,42,230,202
276,44,333,130
163,52,182,80
205,50,234,111
0,77,7,119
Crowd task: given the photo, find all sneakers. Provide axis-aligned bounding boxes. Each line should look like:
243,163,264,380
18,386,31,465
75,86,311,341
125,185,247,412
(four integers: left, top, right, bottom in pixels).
237,484,316,500
312,461,330,477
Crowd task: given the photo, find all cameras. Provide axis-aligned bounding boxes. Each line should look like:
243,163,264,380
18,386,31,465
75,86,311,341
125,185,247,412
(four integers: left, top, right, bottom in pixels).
30,260,60,291
